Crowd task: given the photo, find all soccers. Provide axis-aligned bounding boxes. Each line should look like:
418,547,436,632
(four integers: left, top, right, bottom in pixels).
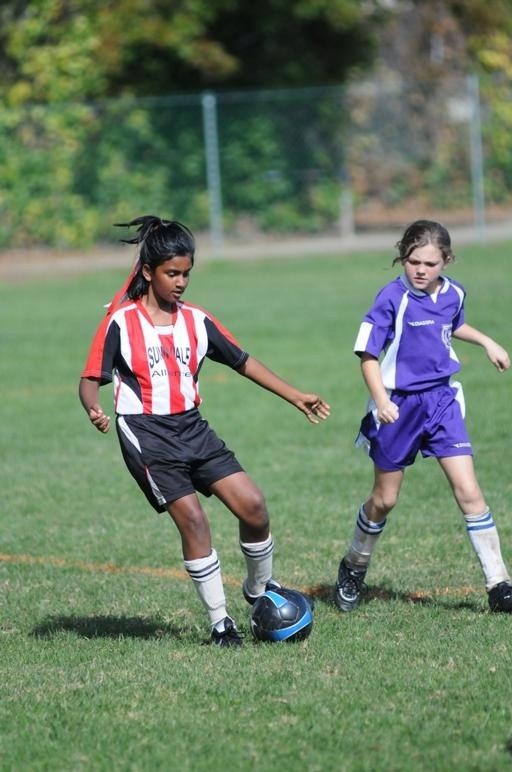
251,588,314,646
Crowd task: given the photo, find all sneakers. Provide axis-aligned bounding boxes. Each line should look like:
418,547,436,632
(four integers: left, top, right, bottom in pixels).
211,616,247,648
242,578,314,612
334,556,368,613
488,581,512,612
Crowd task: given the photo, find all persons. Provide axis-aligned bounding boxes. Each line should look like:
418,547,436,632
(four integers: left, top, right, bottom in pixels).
79,216,332,648
335,220,512,612
